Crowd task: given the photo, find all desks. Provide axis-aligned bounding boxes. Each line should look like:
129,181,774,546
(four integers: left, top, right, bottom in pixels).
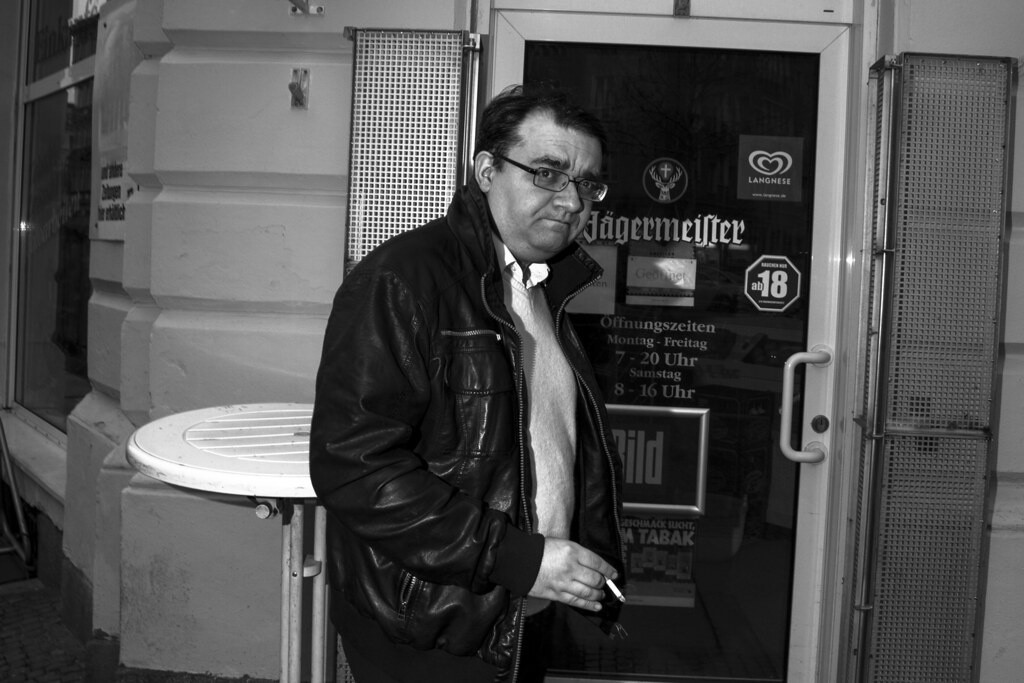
125,401,335,683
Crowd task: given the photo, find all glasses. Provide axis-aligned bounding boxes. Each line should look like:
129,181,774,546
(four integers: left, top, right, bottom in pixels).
498,153,608,202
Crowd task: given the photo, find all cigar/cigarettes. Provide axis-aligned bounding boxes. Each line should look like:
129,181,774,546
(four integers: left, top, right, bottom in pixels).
603,575,626,603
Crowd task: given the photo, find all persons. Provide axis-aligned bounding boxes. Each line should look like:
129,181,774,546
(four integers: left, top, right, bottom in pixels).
307,80,629,683
